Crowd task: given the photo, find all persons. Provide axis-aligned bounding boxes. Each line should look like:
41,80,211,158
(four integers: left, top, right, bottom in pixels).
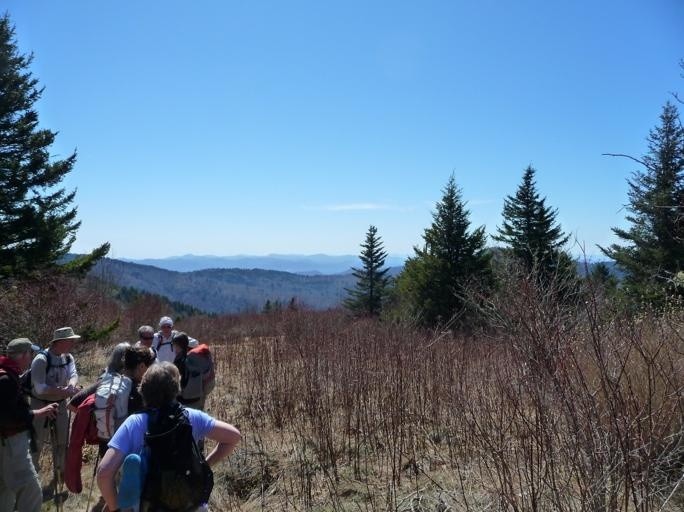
0,337,60,512
30,327,82,496
93,316,241,512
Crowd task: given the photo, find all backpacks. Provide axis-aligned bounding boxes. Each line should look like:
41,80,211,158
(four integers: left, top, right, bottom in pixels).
132,406,215,510
182,342,216,401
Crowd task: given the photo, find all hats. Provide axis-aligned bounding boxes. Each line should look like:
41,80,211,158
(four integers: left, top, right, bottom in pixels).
5,337,41,354
47,326,82,344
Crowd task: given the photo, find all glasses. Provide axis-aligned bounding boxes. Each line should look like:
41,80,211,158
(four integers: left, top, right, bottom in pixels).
141,334,154,341
